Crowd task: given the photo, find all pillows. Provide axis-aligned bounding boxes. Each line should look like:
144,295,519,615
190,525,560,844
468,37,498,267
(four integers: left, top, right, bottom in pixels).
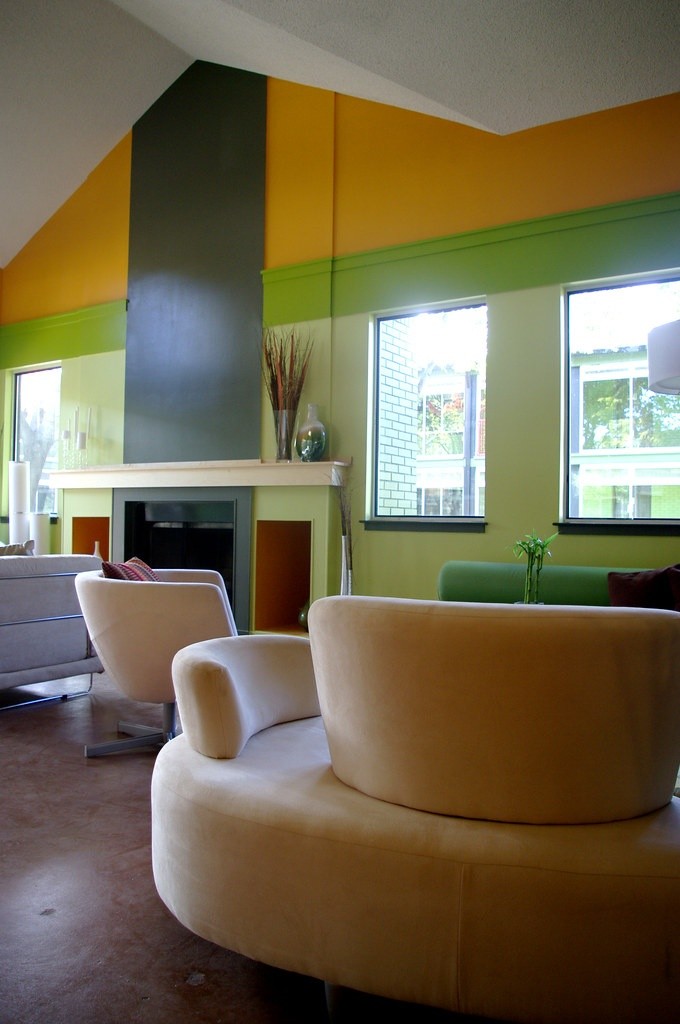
608,564,680,612
102,557,159,581
0,540,34,556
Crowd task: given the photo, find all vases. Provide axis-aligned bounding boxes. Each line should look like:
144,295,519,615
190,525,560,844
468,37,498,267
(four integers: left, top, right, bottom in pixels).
93,541,103,561
296,404,326,463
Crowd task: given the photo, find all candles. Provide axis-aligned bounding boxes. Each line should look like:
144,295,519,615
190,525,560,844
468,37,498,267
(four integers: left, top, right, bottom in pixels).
63,407,92,449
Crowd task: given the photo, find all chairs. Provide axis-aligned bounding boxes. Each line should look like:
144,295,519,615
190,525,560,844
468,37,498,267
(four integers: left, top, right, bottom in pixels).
0,554,105,709
75,569,239,757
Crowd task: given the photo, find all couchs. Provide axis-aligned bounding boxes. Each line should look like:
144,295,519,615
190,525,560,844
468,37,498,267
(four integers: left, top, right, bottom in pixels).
437,561,657,606
151,596,680,1024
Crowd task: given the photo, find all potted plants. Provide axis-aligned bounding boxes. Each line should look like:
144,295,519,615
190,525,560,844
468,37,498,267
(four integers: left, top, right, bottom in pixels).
254,320,316,463
507,527,560,605
320,466,364,596
